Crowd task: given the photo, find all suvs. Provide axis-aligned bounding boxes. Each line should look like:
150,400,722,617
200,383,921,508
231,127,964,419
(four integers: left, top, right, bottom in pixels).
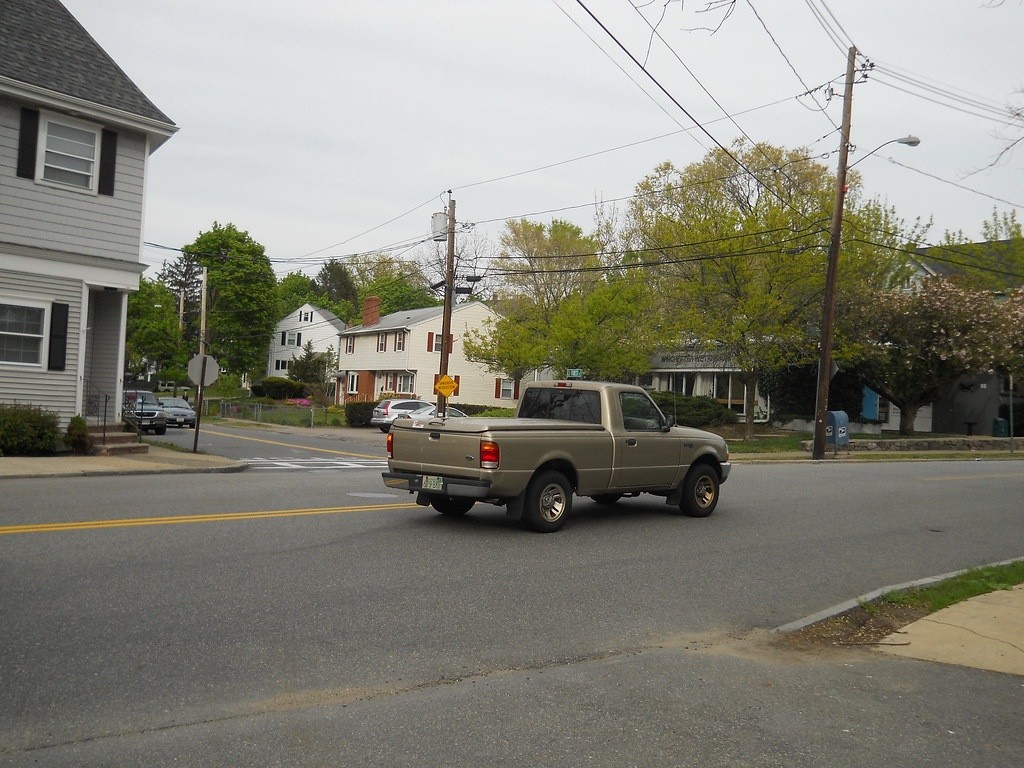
370,398,435,432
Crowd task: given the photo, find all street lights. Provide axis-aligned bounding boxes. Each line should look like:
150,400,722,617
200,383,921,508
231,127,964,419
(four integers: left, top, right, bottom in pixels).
811,134,921,461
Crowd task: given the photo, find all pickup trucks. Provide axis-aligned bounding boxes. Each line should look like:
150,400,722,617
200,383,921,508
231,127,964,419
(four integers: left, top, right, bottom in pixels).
381,377,733,531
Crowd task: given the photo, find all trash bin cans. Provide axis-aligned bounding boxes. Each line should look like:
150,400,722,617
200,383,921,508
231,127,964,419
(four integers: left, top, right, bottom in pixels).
825,411,850,454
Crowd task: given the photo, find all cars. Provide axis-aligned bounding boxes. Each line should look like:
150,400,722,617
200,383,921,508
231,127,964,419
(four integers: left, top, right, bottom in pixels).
398,405,468,420
157,397,197,429
123,390,167,435
